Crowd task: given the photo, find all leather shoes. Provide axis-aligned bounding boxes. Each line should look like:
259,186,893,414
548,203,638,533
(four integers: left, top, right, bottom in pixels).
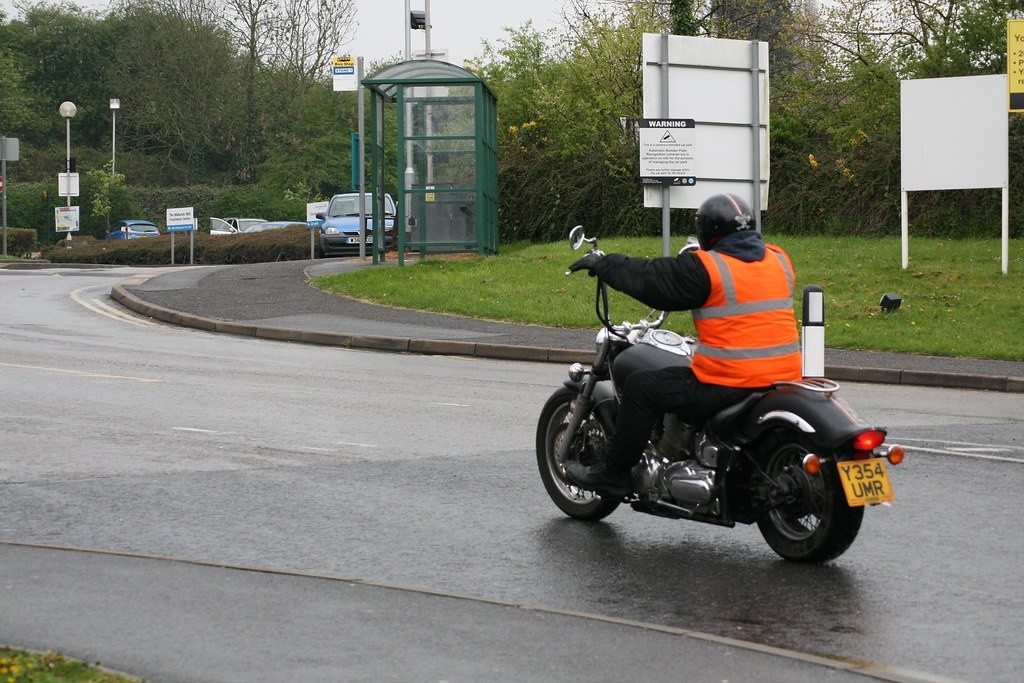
564,461,633,497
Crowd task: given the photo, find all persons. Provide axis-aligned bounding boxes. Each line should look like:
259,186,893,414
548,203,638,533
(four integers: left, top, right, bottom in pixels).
564,194,803,497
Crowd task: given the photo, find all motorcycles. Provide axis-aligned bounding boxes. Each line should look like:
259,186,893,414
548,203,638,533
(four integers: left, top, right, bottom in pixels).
535,224,904,563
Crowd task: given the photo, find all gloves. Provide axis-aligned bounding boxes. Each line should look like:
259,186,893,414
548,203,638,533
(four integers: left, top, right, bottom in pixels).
568,251,603,272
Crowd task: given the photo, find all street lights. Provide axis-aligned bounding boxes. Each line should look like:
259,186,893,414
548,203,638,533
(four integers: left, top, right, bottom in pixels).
58,101,77,252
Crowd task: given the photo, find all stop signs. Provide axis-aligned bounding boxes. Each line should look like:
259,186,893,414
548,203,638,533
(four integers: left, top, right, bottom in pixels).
0,176,3,193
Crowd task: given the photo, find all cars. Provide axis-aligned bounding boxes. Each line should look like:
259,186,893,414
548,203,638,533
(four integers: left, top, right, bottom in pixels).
244,221,305,234
315,192,397,257
105,220,160,240
210,217,269,236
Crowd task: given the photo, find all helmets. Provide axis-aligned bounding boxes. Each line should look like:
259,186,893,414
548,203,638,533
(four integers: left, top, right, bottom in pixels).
695,193,756,252
